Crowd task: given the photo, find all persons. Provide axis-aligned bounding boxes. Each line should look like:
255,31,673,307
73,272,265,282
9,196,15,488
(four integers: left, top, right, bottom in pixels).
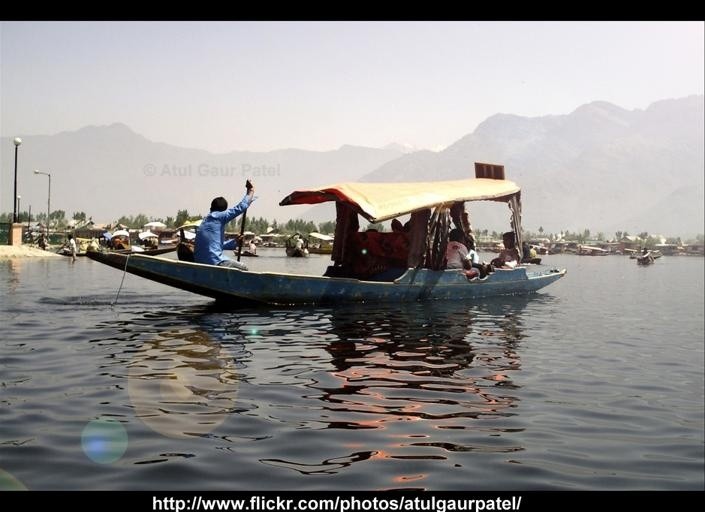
193,177,256,272
522,241,528,257
529,245,536,258
365,218,519,280
641,247,651,265
33,231,51,251
65,233,77,265
177,234,180,243
248,238,256,255
106,235,159,251
296,235,304,249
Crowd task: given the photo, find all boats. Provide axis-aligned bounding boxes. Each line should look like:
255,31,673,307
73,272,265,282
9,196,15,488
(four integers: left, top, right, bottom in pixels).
87,160,568,305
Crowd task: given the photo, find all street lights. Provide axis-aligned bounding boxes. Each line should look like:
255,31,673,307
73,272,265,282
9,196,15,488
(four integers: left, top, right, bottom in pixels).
12,138,21,222
16,195,21,222
33,169,51,239
